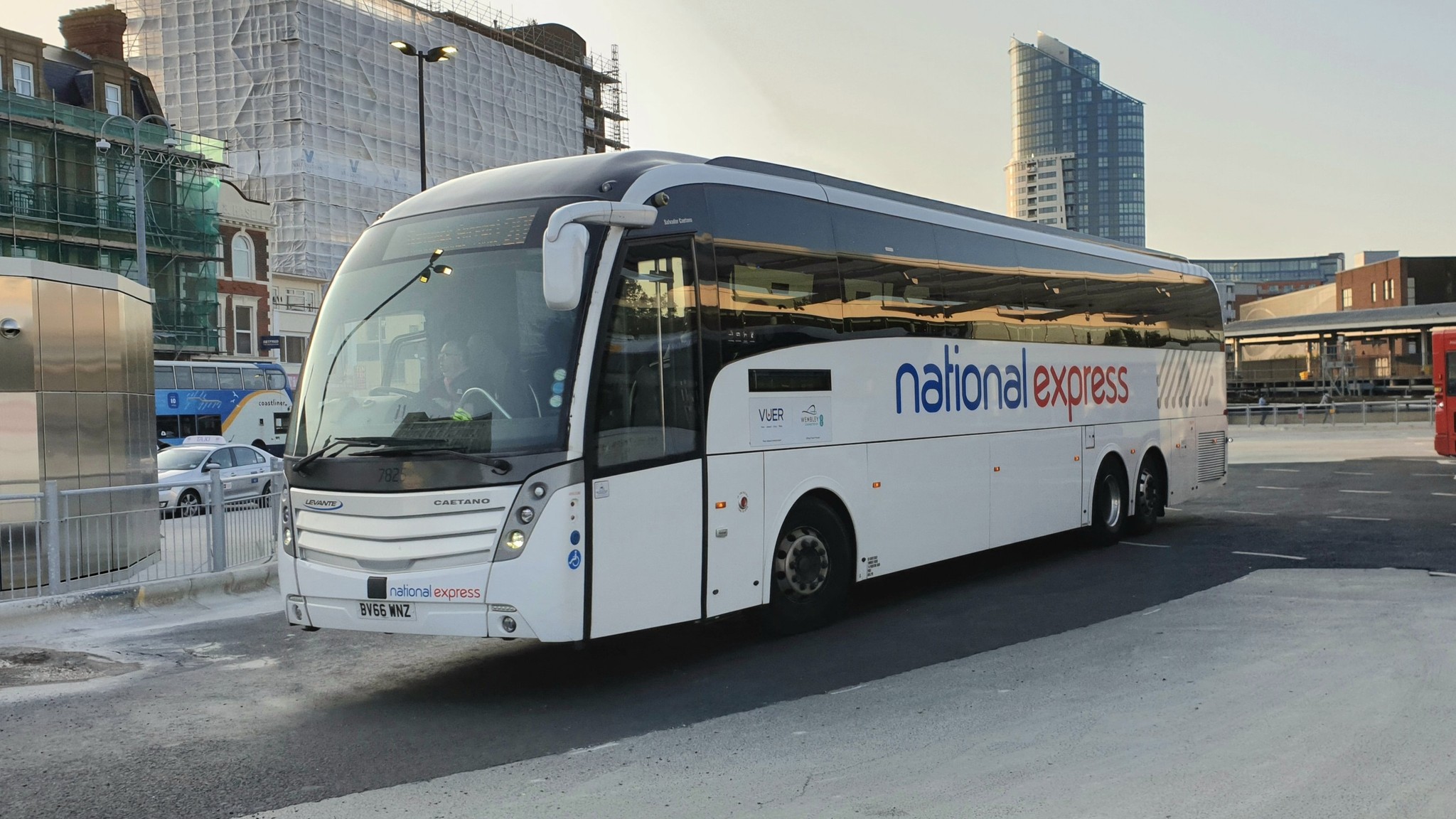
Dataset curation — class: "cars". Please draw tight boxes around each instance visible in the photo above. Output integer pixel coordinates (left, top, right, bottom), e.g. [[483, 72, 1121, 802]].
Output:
[[156, 443, 284, 518], [362, 387, 408, 409]]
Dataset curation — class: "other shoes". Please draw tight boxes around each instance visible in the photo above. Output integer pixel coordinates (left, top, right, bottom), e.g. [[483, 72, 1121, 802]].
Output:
[[1260, 424, 1266, 427]]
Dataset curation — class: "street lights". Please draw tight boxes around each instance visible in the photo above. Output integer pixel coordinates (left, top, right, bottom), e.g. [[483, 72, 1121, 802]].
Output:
[[309, 250, 452, 456], [391, 40, 458, 193]]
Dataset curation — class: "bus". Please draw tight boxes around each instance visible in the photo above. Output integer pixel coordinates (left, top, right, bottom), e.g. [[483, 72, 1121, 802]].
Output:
[[277, 150, 1233, 644], [153, 360, 294, 457], [1431, 326, 1456, 456]]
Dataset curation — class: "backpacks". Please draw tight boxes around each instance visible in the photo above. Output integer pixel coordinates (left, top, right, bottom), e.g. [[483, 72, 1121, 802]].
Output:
[[1324, 394, 1333, 407]]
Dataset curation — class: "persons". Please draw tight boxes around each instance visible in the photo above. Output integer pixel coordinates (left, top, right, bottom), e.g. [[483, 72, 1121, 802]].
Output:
[[1317, 390, 1334, 424], [1258, 393, 1269, 425], [392, 338, 493, 422]]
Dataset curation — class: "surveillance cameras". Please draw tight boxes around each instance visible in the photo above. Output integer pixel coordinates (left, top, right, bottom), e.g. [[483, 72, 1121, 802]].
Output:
[[163, 138, 178, 147], [96, 139, 111, 153]]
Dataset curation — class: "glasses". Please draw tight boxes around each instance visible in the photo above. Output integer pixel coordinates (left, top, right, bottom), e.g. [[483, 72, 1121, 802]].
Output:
[[437, 352, 457, 360]]
